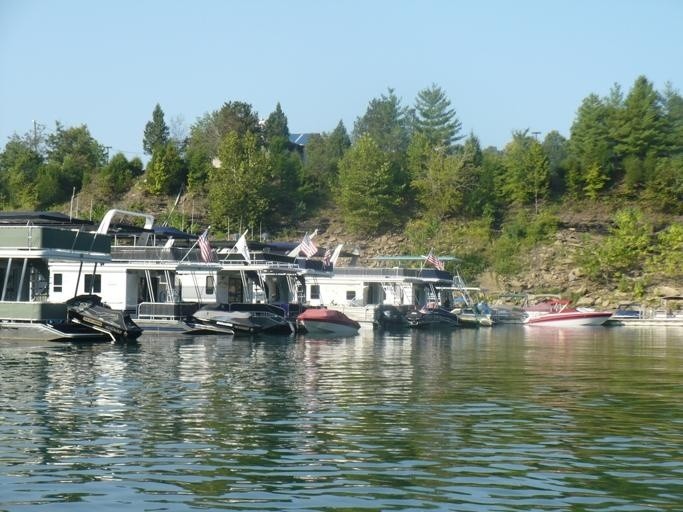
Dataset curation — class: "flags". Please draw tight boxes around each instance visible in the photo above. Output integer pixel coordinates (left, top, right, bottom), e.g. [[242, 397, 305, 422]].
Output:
[[427, 251, 443, 267], [235, 235, 251, 265], [300, 232, 318, 258], [197, 229, 213, 263]]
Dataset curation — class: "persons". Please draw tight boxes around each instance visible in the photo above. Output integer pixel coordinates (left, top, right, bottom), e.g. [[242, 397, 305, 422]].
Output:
[[667, 309, 673, 318], [477, 300, 485, 314]]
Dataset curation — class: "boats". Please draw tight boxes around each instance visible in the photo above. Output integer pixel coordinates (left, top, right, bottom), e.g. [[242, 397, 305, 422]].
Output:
[[492, 293, 527, 323], [373, 268, 497, 333], [620, 295, 683, 327], [516, 297, 613, 330], [295, 308, 361, 337], [0, 188, 310, 344], [604, 294, 647, 329]]
